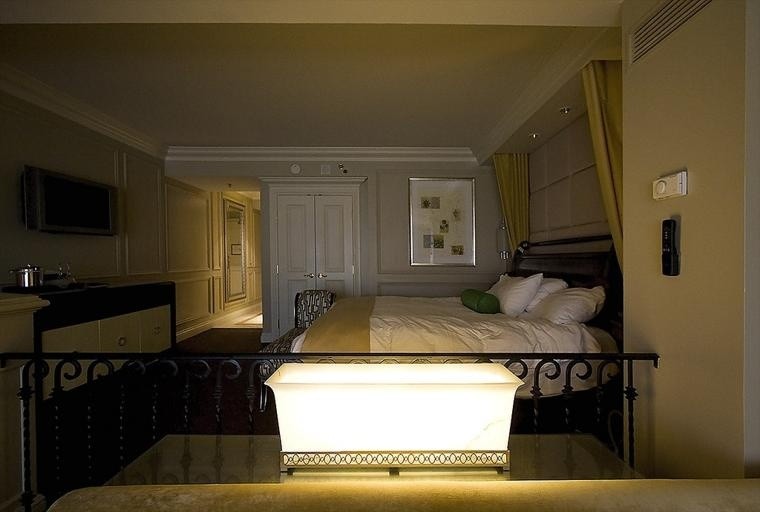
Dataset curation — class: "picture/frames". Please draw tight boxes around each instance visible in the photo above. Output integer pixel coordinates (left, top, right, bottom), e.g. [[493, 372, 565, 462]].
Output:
[[408, 176, 475, 266]]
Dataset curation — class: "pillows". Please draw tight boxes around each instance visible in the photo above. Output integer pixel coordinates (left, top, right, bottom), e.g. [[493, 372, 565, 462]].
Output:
[[528, 285, 606, 321], [460, 287, 496, 310], [526, 276, 567, 310], [485, 273, 543, 318]]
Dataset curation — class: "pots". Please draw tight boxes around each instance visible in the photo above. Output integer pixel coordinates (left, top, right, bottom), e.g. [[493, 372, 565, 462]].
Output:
[[8, 264, 41, 290]]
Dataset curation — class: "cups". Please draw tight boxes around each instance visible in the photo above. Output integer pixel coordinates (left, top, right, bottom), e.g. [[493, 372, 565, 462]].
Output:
[[72, 272, 80, 284]]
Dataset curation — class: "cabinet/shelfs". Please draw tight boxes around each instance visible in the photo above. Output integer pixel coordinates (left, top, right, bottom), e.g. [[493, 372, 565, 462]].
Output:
[[31, 283, 174, 401]]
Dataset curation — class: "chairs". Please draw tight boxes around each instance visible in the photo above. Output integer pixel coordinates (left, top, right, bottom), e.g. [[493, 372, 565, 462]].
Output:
[[292, 290, 336, 329]]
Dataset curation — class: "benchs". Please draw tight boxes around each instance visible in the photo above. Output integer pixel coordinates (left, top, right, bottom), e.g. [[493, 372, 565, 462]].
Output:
[[258, 326, 308, 412]]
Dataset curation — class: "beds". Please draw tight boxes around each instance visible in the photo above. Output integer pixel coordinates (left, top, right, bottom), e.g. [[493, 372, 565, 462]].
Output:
[[294, 233, 622, 421]]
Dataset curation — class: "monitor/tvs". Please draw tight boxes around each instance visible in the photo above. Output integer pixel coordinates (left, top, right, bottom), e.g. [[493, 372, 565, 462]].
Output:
[[20, 164, 120, 237]]
